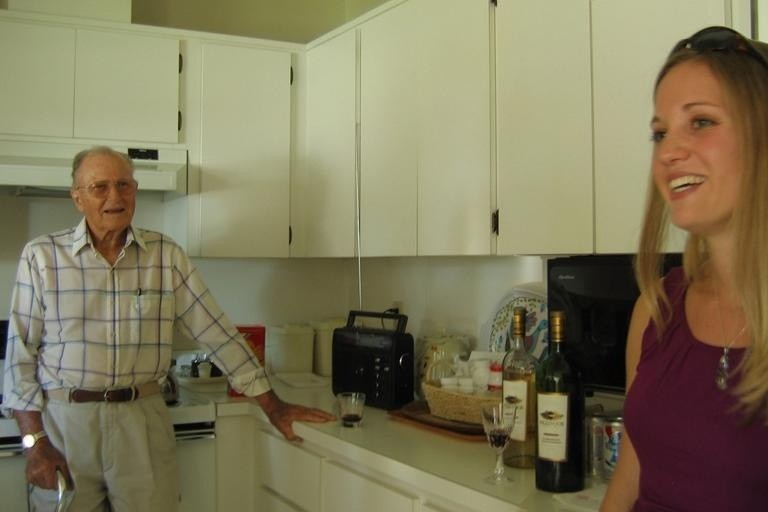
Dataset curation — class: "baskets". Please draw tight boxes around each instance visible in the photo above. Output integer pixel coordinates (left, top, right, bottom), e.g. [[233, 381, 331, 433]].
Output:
[[423, 361, 502, 427]]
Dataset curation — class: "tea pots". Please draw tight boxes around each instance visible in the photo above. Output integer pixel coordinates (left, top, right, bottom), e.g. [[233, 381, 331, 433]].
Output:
[[414, 322, 470, 401]]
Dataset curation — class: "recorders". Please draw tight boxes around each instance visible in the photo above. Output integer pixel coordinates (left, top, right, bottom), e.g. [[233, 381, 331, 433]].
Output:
[[332, 310, 414, 411]]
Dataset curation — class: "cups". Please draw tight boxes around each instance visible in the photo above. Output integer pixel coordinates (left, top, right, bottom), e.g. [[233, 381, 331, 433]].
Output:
[[335, 389, 366, 425]]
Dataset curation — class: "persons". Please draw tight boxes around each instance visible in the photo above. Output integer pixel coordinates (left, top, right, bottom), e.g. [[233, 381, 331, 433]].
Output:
[[0, 146, 337, 512], [590, 25, 768, 512]]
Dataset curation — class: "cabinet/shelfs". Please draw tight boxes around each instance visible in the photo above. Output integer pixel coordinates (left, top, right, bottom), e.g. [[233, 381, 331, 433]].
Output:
[[294, 18, 357, 260], [0, 443, 35, 511], [175, 435, 217, 511], [357, 2, 490, 260], [252, 430, 479, 512], [494, 0, 752, 255], [0, 15, 181, 144], [162, 34, 292, 259]]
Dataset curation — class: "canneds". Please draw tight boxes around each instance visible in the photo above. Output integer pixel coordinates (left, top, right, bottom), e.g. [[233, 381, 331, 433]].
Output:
[[583, 414, 607, 476], [602, 417, 626, 473]]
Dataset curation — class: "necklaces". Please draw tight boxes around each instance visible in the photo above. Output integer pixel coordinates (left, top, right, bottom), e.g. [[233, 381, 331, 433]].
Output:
[[703, 258, 751, 391]]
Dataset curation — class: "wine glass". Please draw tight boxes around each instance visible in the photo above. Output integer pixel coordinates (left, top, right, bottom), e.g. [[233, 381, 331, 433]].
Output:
[[483, 404, 519, 488]]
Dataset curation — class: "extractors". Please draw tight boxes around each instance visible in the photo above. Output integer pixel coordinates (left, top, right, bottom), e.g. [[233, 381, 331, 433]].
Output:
[[0, 0, 188, 195]]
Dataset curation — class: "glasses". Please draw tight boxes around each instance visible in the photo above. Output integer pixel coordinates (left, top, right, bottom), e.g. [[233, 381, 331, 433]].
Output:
[[663, 27, 762, 71], [79, 180, 138, 199]]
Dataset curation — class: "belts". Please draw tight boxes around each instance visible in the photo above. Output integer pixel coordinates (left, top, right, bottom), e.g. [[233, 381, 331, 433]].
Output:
[[41, 380, 161, 403]]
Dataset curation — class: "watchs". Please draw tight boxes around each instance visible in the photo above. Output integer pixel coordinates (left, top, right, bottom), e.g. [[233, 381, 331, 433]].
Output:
[[20, 430, 48, 449]]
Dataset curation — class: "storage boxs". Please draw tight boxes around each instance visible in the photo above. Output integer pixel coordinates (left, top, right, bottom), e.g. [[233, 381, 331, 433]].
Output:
[[270, 322, 315, 376], [313, 317, 366, 378]]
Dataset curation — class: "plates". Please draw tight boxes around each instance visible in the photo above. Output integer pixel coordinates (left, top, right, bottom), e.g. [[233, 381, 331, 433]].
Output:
[[477, 289, 548, 360], [276, 369, 330, 389]]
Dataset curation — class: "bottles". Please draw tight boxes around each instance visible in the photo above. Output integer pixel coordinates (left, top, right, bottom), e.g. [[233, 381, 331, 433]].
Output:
[[188, 350, 224, 379], [536, 309, 585, 494], [441, 359, 501, 395], [501, 303, 538, 466]]
